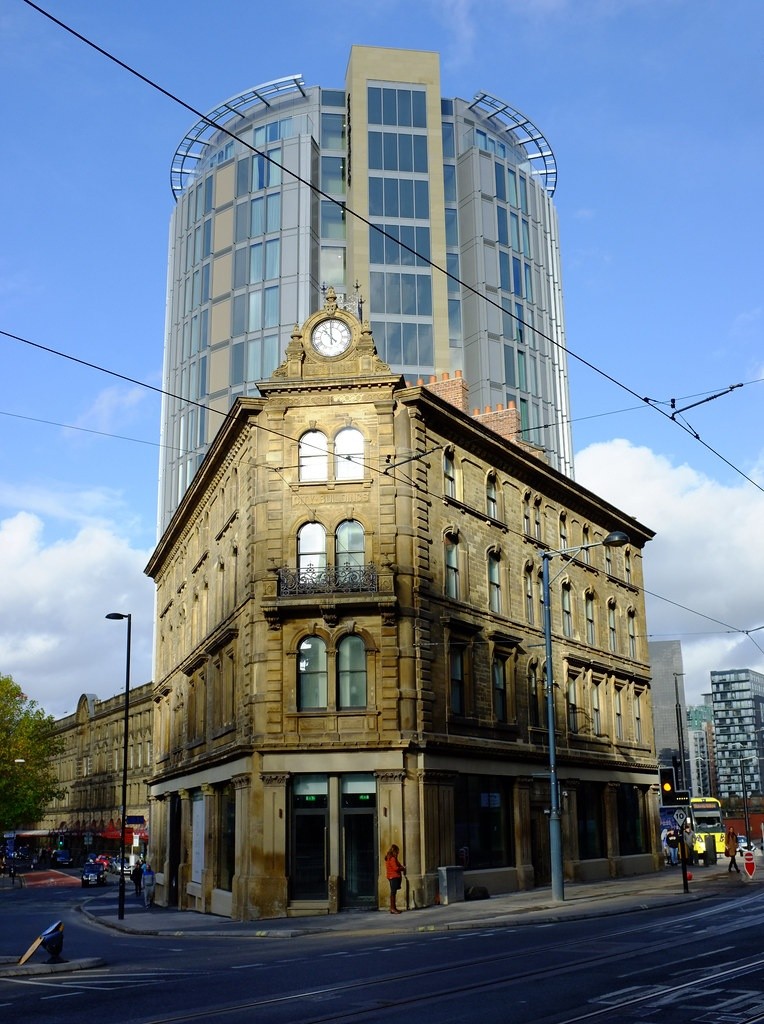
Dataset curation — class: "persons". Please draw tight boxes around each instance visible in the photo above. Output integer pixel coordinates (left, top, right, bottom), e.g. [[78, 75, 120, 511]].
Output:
[[385, 845, 405, 914], [663, 829, 678, 866], [133, 864, 144, 897], [141, 860, 146, 871], [725, 827, 741, 873], [140, 864, 156, 908], [684, 826, 699, 867]]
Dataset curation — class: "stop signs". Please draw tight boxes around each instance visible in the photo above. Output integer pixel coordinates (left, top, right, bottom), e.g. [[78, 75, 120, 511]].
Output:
[[743, 851, 754, 863]]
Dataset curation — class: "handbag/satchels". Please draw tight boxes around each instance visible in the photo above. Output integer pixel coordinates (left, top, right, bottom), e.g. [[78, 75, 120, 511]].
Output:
[[725, 849, 729, 856]]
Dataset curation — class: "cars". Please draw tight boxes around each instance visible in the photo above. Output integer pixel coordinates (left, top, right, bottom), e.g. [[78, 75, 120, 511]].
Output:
[[736, 835, 756, 853], [51, 849, 74, 869], [78, 851, 133, 875], [80, 862, 108, 887]]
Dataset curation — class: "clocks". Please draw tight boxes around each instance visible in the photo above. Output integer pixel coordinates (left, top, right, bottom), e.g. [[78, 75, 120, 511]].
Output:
[[310, 316, 353, 359]]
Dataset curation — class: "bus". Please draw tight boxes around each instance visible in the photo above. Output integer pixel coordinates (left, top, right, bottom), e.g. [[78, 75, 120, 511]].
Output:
[[658, 797, 726, 859]]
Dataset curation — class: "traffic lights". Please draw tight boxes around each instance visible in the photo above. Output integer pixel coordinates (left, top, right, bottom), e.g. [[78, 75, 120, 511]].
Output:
[[658, 767, 692, 807]]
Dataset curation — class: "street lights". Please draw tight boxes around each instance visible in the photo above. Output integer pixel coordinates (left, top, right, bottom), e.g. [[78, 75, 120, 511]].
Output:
[[104, 612, 132, 921], [673, 672, 688, 790], [543, 532, 629, 901], [740, 758, 753, 852]]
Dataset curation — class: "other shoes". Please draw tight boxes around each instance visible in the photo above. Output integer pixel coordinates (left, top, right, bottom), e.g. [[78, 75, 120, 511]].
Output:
[[728, 868, 731, 872], [737, 870, 740, 873]]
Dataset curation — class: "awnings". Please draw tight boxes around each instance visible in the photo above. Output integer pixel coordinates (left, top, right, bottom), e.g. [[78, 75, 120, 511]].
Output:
[[18, 830, 68, 838]]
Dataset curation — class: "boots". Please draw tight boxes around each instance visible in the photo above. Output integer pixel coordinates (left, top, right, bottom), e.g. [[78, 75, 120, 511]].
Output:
[[390, 895, 402, 914]]
